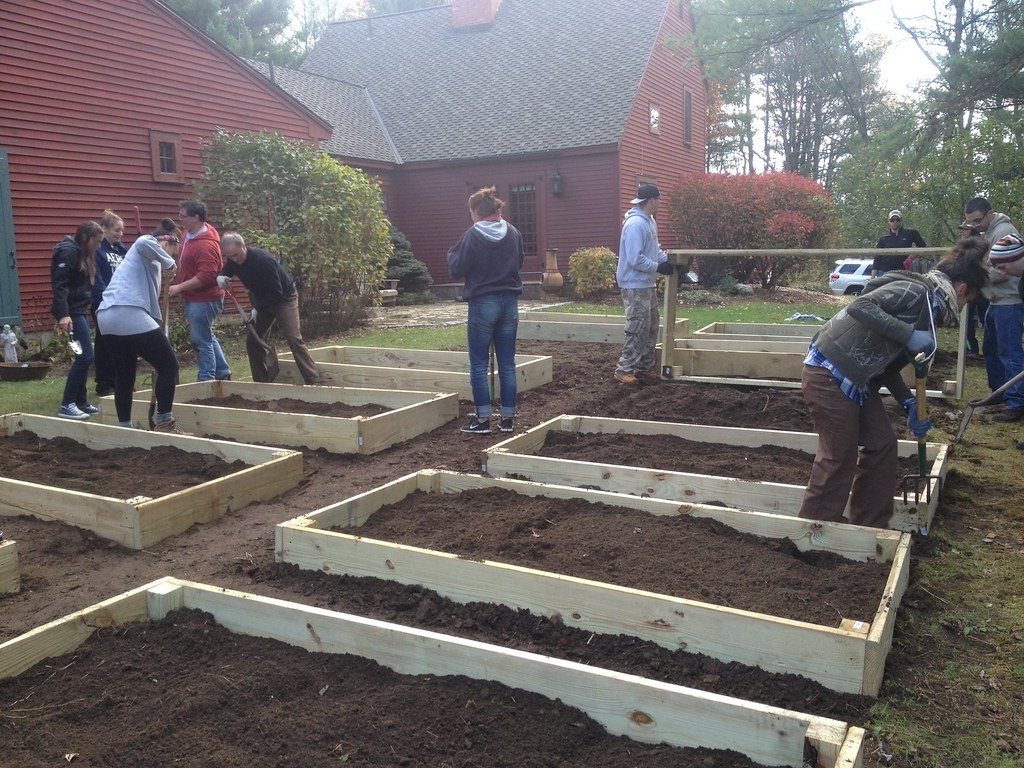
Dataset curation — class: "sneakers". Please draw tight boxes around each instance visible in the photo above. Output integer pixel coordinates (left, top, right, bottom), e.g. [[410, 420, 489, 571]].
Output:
[[154, 417, 195, 436], [460, 415, 492, 433], [497, 416, 513, 436], [58, 403, 90, 419], [80, 404, 99, 415]]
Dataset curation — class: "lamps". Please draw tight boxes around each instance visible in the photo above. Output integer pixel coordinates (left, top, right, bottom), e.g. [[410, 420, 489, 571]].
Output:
[[549, 169, 563, 193]]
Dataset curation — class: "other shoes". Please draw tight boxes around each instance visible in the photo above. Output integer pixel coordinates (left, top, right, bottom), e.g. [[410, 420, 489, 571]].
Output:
[[973, 390, 1005, 405], [966, 349, 979, 359], [614, 370, 638, 384], [95, 381, 115, 396], [634, 368, 657, 379], [992, 407, 1024, 422]]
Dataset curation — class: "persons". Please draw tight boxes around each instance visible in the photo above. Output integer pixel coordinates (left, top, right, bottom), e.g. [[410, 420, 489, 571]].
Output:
[[90, 210, 129, 397], [168, 201, 232, 382], [989, 233, 1024, 450], [965, 197, 1024, 423], [50, 221, 105, 420], [613, 185, 674, 383], [95, 218, 195, 436], [0, 325, 18, 363], [872, 210, 926, 277], [216, 233, 320, 385], [798, 237, 991, 529], [958, 219, 990, 355], [447, 188, 525, 434]]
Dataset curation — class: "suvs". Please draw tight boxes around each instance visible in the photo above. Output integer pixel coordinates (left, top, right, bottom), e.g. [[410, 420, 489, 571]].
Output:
[[826, 257, 876, 298]]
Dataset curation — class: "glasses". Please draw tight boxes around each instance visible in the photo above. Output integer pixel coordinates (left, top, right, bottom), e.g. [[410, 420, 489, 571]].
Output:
[[967, 209, 991, 224], [891, 218, 900, 222], [180, 214, 191, 220]]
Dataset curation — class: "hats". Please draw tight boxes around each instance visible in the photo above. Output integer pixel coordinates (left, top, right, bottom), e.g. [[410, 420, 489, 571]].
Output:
[[630, 184, 660, 204], [989, 232, 1024, 268], [958, 219, 973, 230], [888, 210, 901, 220]]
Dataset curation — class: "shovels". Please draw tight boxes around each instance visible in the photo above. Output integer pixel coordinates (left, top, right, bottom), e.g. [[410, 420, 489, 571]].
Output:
[[226, 288, 280, 383], [148, 266, 173, 431], [957, 370, 1024, 442], [67, 331, 82, 355]]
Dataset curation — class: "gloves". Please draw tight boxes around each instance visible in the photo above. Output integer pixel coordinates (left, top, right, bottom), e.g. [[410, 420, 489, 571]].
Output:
[[908, 330, 934, 358], [656, 260, 682, 275], [903, 256, 913, 270], [244, 309, 258, 325], [217, 276, 231, 290], [902, 396, 930, 436]]
